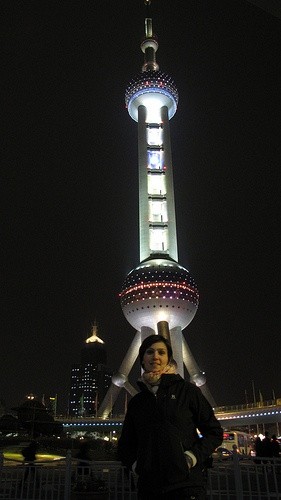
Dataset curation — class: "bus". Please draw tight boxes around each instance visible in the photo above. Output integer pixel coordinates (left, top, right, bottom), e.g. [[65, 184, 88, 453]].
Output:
[[216, 431, 256, 457]]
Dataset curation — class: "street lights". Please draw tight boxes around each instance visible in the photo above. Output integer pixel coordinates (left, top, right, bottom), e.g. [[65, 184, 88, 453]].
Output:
[[25, 392, 37, 438]]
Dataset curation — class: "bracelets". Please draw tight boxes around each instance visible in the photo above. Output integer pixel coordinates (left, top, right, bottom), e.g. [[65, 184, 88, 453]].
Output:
[[185, 454, 193, 466]]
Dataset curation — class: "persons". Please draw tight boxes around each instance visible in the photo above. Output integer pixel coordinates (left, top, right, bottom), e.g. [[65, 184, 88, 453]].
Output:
[[254, 431, 280, 472], [73, 441, 95, 490], [18, 439, 41, 492], [118, 334, 224, 500]]
